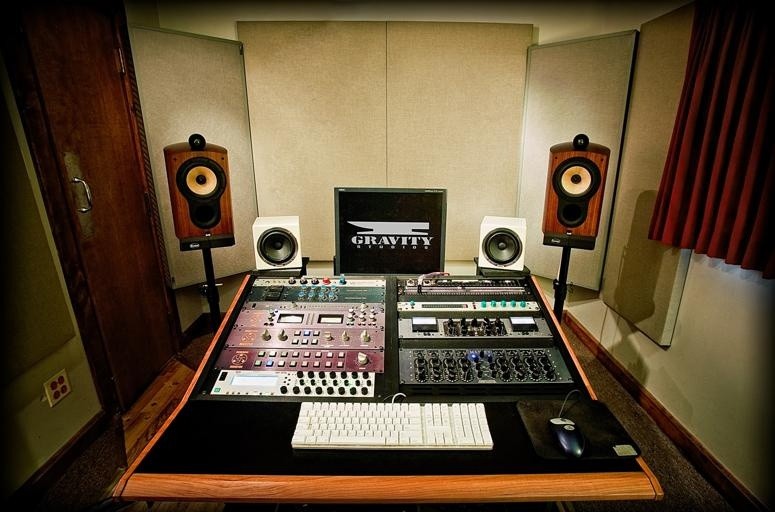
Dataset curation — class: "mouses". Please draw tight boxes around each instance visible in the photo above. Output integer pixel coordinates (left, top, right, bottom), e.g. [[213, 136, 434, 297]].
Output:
[[549, 417, 585, 457]]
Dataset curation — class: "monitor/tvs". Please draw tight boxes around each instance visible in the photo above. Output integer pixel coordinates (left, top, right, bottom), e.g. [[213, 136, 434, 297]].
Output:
[[334, 187, 447, 276]]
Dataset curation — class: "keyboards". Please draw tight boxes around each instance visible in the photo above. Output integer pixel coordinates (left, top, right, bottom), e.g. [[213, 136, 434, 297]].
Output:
[[291, 402, 495, 451]]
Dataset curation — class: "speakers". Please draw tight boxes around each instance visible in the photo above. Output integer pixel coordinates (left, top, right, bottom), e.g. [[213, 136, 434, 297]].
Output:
[[252, 216, 303, 270], [478, 216, 526, 271], [542, 143, 611, 237], [163, 142, 234, 240]]
